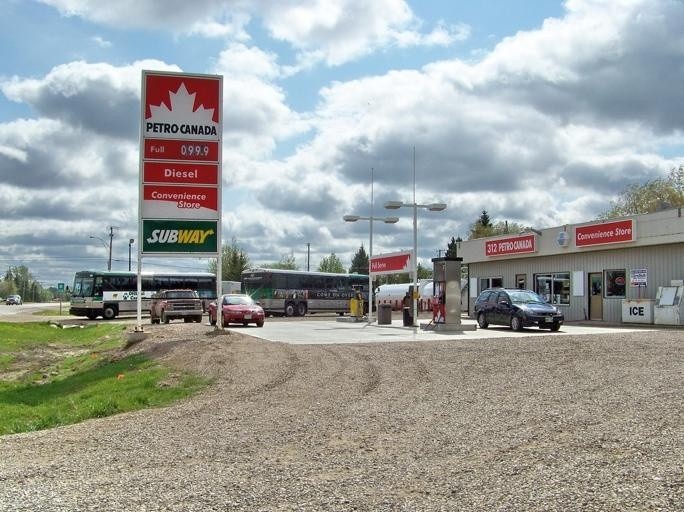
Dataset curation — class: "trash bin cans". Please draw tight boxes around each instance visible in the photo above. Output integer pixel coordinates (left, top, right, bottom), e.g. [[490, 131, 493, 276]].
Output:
[[378, 303, 391, 324]]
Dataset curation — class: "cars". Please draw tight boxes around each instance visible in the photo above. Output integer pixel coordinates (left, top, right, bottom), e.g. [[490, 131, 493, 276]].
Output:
[[473, 287, 564, 331], [6, 294, 22, 305], [208, 293, 265, 327]]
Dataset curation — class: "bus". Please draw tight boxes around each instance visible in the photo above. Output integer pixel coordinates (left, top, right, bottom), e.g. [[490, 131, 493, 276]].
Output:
[[240, 268, 376, 318], [66, 271, 217, 319]]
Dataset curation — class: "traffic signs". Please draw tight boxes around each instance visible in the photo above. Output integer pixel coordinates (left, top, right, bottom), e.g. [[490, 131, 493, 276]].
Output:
[[58, 283, 64, 293]]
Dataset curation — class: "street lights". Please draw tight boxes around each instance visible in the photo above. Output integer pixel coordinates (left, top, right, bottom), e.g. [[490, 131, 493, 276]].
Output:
[[342, 214, 398, 321], [89, 226, 114, 271], [384, 200, 447, 324], [128, 239, 135, 271]]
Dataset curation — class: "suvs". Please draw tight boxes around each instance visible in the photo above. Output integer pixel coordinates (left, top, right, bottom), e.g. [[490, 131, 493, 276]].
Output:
[[151, 288, 204, 324]]
